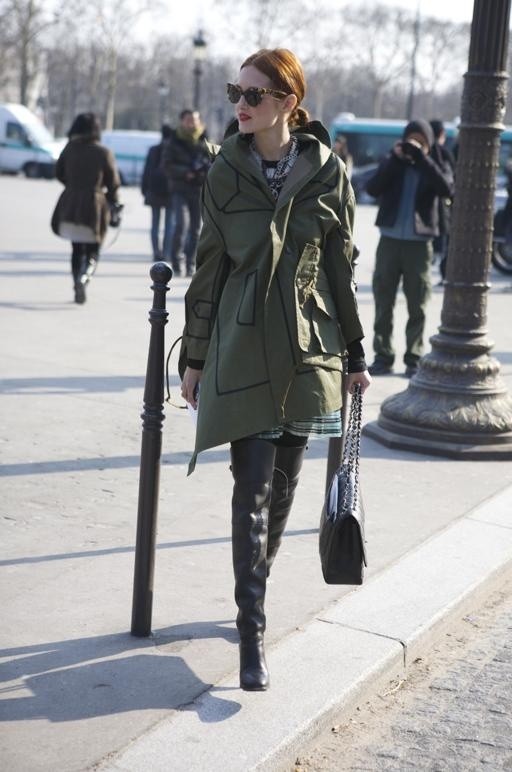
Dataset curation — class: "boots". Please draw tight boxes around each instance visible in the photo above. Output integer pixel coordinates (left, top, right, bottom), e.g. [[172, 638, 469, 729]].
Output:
[[71, 254, 96, 304], [231, 440, 307, 692]]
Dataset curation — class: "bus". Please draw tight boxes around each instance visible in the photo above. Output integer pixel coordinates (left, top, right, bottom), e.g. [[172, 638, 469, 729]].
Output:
[[327, 112, 511, 207]]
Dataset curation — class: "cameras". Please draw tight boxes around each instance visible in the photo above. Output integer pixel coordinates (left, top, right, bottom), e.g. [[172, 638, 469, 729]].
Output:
[[402, 143, 416, 155]]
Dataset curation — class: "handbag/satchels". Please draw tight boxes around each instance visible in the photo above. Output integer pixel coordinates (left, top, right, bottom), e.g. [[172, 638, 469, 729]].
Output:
[[318, 461, 366, 585]]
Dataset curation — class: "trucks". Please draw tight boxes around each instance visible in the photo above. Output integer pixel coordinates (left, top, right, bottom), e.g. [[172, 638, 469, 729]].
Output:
[[1, 102, 60, 180], [94, 128, 167, 187]]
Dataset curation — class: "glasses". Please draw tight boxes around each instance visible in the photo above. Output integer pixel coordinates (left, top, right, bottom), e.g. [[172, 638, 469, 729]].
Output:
[[225, 81, 287, 107]]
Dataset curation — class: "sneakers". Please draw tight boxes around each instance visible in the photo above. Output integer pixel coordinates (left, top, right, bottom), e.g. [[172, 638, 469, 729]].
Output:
[[369, 361, 395, 375]]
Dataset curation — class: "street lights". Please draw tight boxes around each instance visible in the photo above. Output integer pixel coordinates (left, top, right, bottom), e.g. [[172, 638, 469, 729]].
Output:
[[191, 28, 208, 115]]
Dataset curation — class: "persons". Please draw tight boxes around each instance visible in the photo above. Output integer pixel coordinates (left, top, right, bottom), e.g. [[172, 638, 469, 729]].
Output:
[[162, 107, 212, 278], [140, 125, 182, 268], [49, 111, 120, 306], [176, 46, 375, 691], [425, 115, 451, 288], [362, 116, 454, 380], [331, 132, 353, 190]]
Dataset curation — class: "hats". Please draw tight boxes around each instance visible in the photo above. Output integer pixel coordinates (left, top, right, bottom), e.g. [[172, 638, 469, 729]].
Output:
[[402, 118, 434, 154]]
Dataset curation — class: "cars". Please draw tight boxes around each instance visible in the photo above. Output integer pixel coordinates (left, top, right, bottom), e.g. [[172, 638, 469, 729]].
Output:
[[490, 171, 511, 278]]
